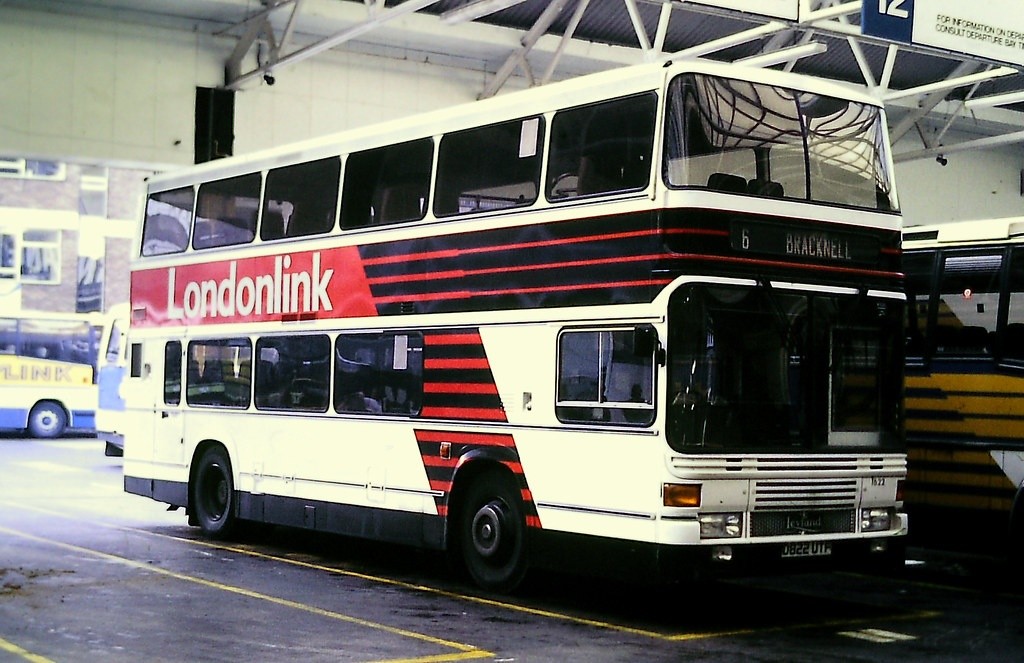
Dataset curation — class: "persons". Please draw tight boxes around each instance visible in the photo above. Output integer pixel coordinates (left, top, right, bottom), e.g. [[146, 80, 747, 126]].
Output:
[[338, 373, 382, 413]]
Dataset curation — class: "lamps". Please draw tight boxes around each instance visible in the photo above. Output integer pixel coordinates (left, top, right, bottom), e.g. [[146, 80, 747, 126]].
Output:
[[264, 71, 275, 85], [936, 153, 948, 166]]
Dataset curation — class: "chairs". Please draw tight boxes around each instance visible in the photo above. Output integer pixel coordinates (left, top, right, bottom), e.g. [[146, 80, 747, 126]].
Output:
[[172, 359, 420, 417], [146, 158, 783, 252], [930, 322, 1024, 362]]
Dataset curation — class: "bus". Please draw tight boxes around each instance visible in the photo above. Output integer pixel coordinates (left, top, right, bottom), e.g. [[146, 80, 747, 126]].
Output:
[[830, 215, 1024, 574], [97, 301, 129, 458], [121, 51, 916, 601], [0, 309, 107, 440]]
